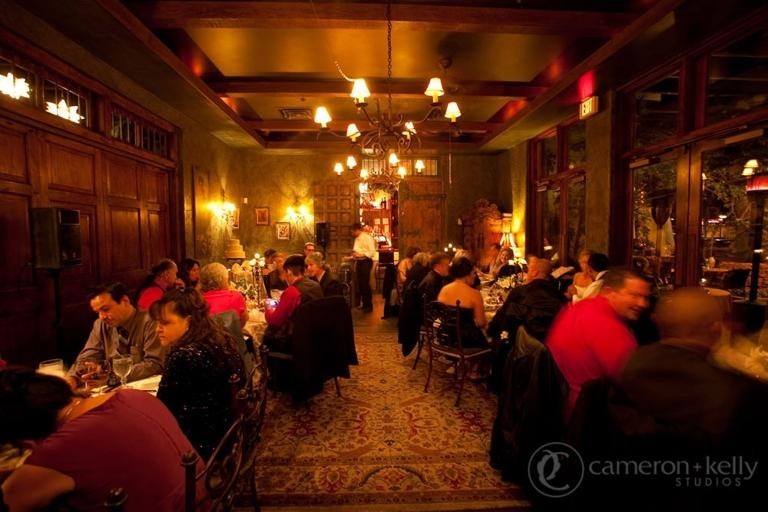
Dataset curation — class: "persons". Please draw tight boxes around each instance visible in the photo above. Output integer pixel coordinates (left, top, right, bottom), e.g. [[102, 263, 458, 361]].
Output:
[[342, 224, 375, 315]]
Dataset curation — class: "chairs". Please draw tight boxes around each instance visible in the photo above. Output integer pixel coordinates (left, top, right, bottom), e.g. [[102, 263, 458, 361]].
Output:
[[390, 254, 565, 482], [0, 254, 356, 509]]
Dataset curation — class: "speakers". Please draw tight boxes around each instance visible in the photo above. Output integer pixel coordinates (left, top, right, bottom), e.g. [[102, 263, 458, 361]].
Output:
[[316, 222, 330, 246], [32, 207, 82, 270]]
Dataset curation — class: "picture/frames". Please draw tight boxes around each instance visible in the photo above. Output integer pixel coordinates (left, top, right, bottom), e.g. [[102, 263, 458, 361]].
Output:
[[254, 207, 270, 227], [274, 221, 291, 241], [192, 164, 210, 260]]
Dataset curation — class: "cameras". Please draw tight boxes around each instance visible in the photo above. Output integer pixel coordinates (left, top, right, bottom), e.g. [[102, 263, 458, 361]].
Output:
[[269, 299, 279, 308]]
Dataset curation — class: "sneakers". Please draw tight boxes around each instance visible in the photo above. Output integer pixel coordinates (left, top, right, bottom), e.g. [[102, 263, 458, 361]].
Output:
[[437, 356, 452, 365]]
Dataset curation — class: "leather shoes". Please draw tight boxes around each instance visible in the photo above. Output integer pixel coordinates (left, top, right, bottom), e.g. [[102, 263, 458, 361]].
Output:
[[358, 306, 373, 312]]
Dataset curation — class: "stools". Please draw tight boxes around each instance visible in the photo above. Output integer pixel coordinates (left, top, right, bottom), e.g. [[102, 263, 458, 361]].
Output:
[[373, 262, 393, 291]]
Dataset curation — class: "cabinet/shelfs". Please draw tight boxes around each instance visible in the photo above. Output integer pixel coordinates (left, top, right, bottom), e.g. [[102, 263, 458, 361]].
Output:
[[471, 207, 504, 269]]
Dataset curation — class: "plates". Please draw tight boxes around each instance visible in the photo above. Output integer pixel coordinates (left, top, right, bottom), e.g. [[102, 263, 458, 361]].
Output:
[[483, 297, 504, 306]]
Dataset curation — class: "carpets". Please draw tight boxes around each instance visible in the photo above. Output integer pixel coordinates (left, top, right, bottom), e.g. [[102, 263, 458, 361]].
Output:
[[222, 331, 544, 510]]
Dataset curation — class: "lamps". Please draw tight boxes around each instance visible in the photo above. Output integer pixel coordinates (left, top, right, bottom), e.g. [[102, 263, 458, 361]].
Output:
[[307, 0, 462, 207]]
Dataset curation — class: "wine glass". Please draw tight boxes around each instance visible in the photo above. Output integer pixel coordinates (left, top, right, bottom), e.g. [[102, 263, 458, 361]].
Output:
[[40, 354, 134, 394]]
